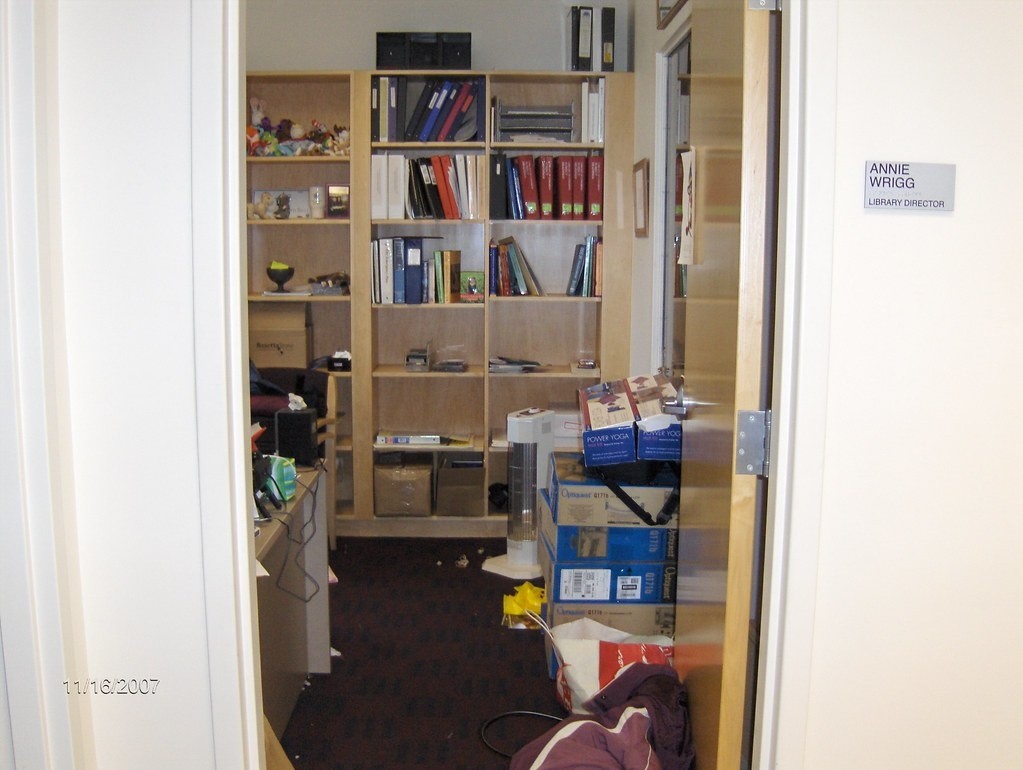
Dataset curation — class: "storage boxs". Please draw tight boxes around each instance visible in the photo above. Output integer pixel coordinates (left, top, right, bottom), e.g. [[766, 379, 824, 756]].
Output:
[[248, 302, 313, 369], [539, 373, 683, 681], [436, 454, 485, 516], [374, 452, 433, 516]]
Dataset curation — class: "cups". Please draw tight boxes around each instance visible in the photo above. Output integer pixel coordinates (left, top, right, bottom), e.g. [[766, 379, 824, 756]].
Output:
[[309, 186, 325, 219]]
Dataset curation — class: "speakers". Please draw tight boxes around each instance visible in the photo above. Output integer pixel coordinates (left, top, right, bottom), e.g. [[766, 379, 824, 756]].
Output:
[[274, 408, 318, 468]]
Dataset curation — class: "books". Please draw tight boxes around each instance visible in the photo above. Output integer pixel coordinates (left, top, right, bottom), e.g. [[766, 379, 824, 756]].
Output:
[[371, 74, 486, 142], [261, 291, 313, 297], [488, 355, 541, 374], [489, 428, 509, 448], [371, 236, 484, 304], [488, 235, 541, 297], [377, 450, 406, 469], [579, 374, 677, 431], [490, 76, 605, 144], [561, 5, 616, 72], [566, 235, 603, 298], [373, 430, 475, 450], [451, 460, 483, 468], [489, 149, 604, 221], [371, 154, 485, 219]]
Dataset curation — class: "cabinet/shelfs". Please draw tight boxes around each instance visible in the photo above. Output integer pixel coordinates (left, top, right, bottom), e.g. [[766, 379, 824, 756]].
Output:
[[245, 67, 636, 538]]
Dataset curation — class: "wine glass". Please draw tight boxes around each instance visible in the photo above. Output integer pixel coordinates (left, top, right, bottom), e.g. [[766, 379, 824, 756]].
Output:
[[266, 267, 294, 293]]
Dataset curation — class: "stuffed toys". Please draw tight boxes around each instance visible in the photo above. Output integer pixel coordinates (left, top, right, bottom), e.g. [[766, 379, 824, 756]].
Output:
[[246, 191, 291, 220], [245, 97, 350, 157]]
